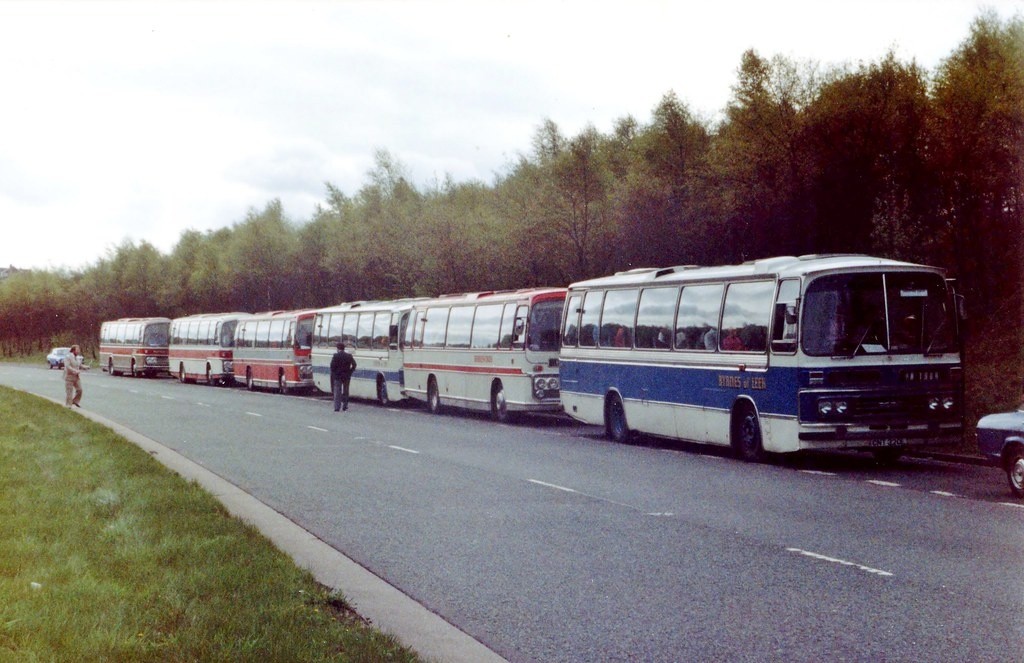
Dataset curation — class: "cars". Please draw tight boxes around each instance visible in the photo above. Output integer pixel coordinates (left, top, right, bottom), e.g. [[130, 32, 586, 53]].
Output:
[[47, 347, 84, 370], [974, 410, 1024, 499]]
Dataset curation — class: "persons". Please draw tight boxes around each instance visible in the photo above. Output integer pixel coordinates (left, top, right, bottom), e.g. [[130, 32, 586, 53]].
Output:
[[593, 321, 746, 352], [64, 344, 88, 407], [329, 343, 357, 411]]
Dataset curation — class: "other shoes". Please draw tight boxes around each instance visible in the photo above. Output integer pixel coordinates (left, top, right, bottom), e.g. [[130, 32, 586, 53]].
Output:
[[343, 407, 349, 410], [335, 408, 339, 411], [72, 402, 80, 407]]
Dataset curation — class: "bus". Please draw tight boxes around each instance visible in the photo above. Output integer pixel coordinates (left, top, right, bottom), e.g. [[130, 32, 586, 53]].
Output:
[[100, 317, 172, 379], [168, 312, 252, 387], [232, 307, 320, 395], [403, 286, 570, 424], [311, 297, 430, 408], [557, 252, 971, 465]]
